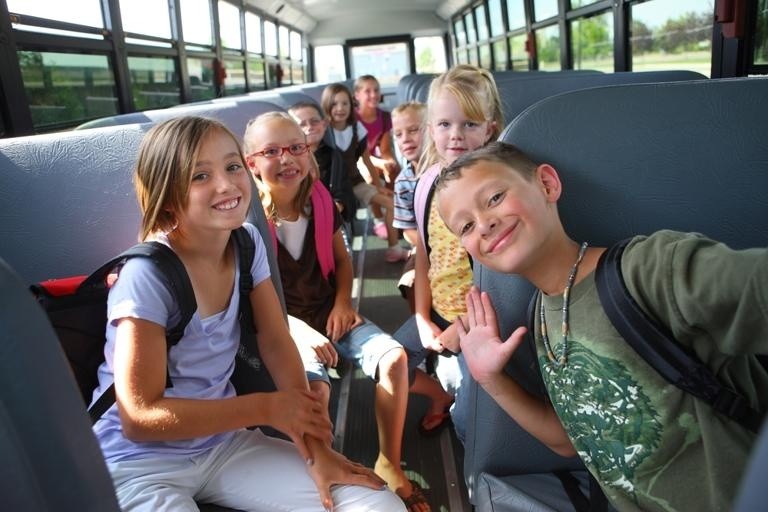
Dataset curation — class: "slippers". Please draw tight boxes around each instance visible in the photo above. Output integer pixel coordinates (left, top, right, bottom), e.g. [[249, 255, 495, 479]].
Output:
[[394, 479, 432, 512], [421, 405, 451, 435]]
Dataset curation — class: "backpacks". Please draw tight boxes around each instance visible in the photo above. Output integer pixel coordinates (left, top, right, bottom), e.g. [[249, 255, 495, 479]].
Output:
[[23, 226, 255, 432]]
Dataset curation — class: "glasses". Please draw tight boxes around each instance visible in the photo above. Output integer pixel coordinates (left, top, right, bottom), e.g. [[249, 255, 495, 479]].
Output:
[[246, 142, 309, 158]]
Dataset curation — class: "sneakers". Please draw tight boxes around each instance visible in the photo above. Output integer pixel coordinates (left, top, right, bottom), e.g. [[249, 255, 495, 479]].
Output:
[[385, 245, 414, 263], [374, 220, 391, 240]]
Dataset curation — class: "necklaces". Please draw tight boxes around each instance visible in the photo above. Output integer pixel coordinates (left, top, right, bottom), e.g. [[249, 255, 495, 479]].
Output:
[[539, 241, 588, 367]]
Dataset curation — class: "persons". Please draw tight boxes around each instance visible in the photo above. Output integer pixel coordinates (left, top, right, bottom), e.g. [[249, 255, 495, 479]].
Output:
[[434, 140, 766, 511], [84, 115, 408, 512], [390, 100, 426, 315], [320, 82, 410, 264], [351, 75, 401, 240], [391, 62, 506, 434], [287, 103, 358, 222], [241, 110, 433, 512]]
